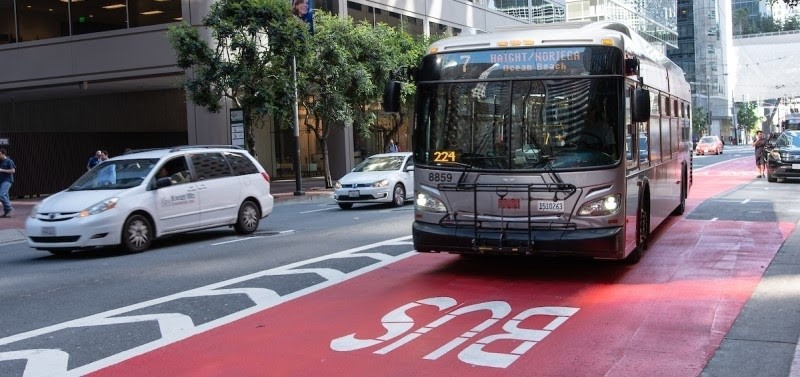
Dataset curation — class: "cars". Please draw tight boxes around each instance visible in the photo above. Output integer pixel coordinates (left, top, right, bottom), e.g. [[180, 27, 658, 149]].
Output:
[[765, 130, 800, 182], [334, 151, 415, 210], [695, 134, 724, 155]]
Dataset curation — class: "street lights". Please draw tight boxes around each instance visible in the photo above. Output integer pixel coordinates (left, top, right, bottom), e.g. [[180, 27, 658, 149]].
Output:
[[708, 73, 729, 137]]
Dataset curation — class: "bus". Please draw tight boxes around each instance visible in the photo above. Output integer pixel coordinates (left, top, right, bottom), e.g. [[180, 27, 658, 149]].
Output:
[[382, 19, 693, 266], [782, 113, 800, 131]]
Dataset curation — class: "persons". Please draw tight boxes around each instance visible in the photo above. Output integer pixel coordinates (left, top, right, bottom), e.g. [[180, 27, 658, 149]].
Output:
[[152, 165, 169, 187], [387, 139, 401, 153], [572, 96, 616, 156], [292, 0, 308, 21], [0, 149, 16, 218], [87, 149, 116, 185], [753, 131, 767, 178]]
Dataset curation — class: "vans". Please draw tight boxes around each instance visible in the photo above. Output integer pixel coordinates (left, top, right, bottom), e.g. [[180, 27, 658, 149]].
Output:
[[24, 144, 273, 254]]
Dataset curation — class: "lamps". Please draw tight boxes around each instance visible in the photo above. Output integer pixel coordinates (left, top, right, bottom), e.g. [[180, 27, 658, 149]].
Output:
[[80, 81, 88, 96]]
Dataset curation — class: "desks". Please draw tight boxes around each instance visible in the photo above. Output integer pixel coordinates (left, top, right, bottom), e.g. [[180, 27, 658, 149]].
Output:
[[301, 164, 318, 176]]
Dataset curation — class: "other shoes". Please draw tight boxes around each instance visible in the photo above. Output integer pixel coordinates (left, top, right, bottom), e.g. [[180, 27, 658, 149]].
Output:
[[756, 175, 761, 178], [762, 175, 765, 179], [7, 209, 15, 217], [0, 214, 8, 218]]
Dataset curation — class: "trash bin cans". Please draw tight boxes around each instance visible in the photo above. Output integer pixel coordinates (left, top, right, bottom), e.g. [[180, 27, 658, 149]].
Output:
[[733, 138, 736, 145]]
